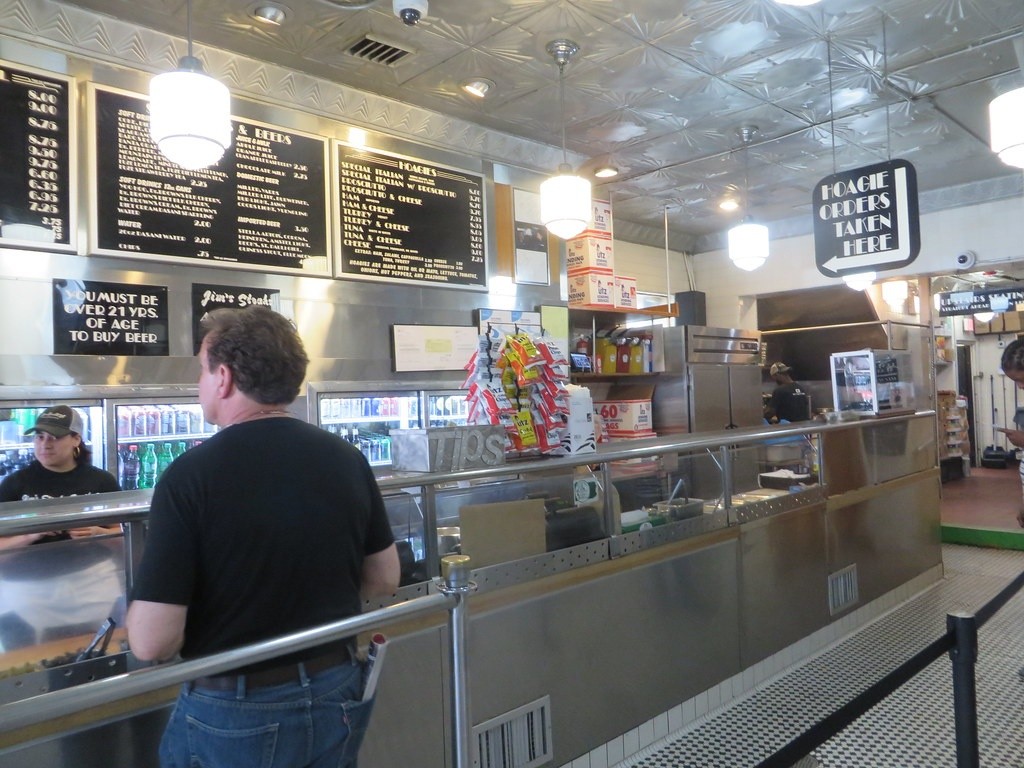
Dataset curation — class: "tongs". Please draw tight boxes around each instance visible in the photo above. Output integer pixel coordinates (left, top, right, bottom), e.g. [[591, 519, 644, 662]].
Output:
[[75, 618, 116, 665], [667, 479, 690, 507]]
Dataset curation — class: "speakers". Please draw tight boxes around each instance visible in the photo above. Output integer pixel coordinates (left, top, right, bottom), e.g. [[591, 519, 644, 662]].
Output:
[[675, 291, 707, 327]]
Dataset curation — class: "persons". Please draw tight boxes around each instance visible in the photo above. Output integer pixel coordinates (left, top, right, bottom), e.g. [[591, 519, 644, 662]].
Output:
[[125, 306, 403, 768], [763, 362, 809, 421], [1001, 340, 1024, 681], [0, 406, 123, 548]]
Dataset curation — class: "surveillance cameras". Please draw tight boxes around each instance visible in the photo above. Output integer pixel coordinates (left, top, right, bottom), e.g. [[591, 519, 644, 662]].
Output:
[[393, 0, 429, 26], [955, 251, 975, 270]]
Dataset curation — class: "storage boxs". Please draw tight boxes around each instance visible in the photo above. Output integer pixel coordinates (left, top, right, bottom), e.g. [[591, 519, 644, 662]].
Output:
[[566, 199, 638, 311], [652, 498, 703, 524], [601, 432, 658, 480], [990, 312, 1004, 333], [973, 313, 990, 334], [590, 383, 655, 436], [1004, 311, 1024, 332]]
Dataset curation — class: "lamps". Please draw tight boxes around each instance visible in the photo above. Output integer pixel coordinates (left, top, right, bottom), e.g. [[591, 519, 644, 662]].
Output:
[[728, 125, 769, 271], [988, 86, 1024, 168], [538, 39, 591, 239], [146, 0, 232, 171]]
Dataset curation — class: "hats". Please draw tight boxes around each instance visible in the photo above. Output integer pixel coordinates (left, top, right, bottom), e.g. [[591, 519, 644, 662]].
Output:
[[770, 362, 792, 375], [25, 405, 85, 439]]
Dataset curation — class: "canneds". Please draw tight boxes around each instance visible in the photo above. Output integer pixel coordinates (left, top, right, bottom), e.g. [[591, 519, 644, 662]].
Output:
[[320, 398, 419, 463], [117, 405, 217, 437], [431, 396, 473, 426]]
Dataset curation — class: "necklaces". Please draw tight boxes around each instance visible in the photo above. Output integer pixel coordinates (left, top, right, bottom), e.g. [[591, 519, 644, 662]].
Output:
[[243, 409, 289, 417]]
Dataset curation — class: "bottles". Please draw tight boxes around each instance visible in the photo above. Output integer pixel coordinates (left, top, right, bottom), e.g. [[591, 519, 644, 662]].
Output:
[[319, 425, 362, 458], [1, 440, 216, 502]]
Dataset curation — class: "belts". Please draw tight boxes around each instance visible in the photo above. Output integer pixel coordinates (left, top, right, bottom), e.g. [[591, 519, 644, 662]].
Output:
[[192, 636, 358, 692]]
